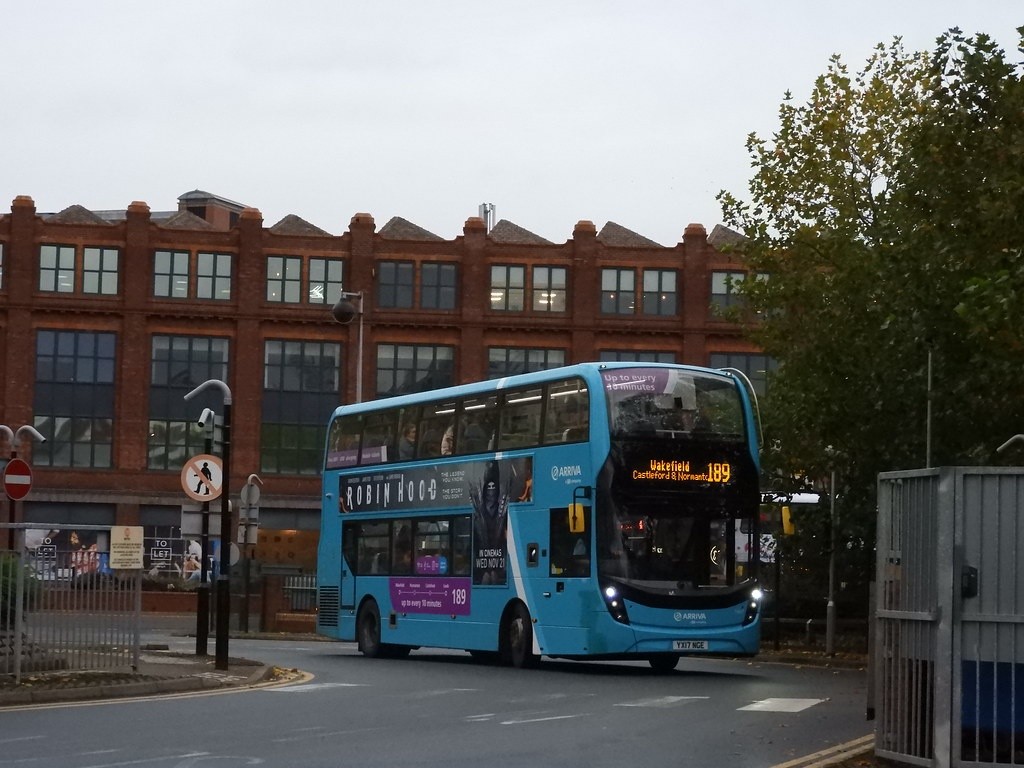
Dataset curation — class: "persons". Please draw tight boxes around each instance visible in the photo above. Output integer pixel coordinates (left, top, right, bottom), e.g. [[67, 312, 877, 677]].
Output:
[[184, 552, 211, 582], [398, 423, 416, 460], [393, 545, 412, 575], [25, 528, 100, 578], [440, 413, 468, 455]]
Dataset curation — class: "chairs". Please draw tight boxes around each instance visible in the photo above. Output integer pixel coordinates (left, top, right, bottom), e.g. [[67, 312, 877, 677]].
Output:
[[370, 552, 477, 577], [369, 422, 580, 465]]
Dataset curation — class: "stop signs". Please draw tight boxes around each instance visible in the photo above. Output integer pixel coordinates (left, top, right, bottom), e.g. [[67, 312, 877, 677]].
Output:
[[2, 458, 33, 501]]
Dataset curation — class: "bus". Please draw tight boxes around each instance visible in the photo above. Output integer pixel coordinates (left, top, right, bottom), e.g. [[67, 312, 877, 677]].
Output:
[[314, 358, 764, 674]]
